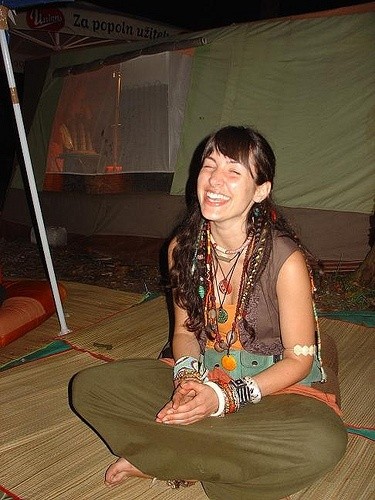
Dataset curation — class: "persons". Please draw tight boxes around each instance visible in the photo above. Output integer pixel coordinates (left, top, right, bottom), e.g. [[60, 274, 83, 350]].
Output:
[[72, 126, 348, 500]]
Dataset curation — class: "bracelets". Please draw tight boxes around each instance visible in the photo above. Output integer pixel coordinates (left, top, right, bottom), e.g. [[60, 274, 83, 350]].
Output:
[[205, 376, 262, 418], [173, 356, 205, 390]]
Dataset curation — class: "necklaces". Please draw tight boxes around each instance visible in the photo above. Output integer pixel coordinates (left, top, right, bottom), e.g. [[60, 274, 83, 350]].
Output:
[[204, 223, 256, 369]]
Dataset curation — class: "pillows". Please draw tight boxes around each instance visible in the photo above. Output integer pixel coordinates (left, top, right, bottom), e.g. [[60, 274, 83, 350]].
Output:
[[0, 276, 68, 348]]
